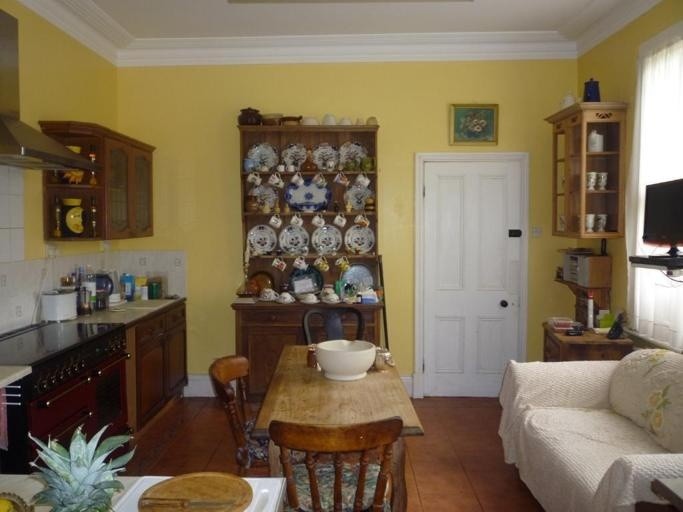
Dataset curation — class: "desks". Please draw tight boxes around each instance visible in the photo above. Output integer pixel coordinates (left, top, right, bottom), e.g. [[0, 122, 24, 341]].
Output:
[[250, 344, 422, 512]]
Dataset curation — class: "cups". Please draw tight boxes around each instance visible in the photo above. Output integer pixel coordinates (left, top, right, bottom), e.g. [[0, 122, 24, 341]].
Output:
[[258, 287, 339, 303], [238, 107, 377, 126], [246, 171, 371, 188], [271, 256, 351, 273], [269, 211, 369, 228], [276, 164, 296, 173], [586, 213, 608, 232]]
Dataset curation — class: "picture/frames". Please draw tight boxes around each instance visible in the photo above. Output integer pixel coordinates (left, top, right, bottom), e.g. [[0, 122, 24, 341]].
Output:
[[447, 103, 499, 146]]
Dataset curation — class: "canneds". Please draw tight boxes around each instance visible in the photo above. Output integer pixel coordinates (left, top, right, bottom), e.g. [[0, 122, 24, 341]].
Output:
[[307, 349, 316, 367], [96, 289, 106, 310], [140, 285, 148, 301]]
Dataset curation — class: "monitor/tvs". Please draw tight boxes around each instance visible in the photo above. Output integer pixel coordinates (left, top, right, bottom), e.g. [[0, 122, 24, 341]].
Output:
[[643, 178, 683, 258]]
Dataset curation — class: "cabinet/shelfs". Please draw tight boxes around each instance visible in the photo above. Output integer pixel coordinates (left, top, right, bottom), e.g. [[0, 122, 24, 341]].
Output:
[[545, 102, 624, 240], [543, 325, 633, 363], [230, 297, 385, 399], [40, 122, 154, 239], [52, 298, 187, 439], [236, 124, 380, 292]]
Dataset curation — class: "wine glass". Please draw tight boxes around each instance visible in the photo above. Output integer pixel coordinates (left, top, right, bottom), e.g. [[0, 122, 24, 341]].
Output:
[[584, 171, 608, 191]]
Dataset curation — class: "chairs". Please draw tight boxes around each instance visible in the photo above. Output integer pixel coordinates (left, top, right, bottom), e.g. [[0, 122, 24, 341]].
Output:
[[265, 415, 406, 512], [208, 356, 269, 478], [303, 308, 364, 345]]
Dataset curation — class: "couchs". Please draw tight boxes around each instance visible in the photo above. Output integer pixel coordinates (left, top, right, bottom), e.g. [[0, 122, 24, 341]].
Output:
[[497, 349, 682, 512]]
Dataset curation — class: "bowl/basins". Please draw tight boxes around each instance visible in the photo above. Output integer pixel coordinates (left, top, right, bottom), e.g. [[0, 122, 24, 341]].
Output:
[[315, 340, 377, 382]]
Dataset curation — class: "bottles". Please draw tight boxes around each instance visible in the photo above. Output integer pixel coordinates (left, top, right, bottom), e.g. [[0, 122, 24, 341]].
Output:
[[86, 196, 98, 238], [306, 344, 317, 367], [88, 143, 100, 185], [51, 194, 61, 239]]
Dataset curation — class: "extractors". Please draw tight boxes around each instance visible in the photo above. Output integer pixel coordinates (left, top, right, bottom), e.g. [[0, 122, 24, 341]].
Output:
[[1, 9, 104, 174]]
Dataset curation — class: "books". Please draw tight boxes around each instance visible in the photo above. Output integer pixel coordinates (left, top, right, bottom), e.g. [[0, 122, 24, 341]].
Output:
[[548, 317, 574, 333]]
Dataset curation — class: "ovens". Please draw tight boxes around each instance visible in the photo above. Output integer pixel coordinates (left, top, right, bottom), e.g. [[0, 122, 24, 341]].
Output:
[[23, 352, 132, 463]]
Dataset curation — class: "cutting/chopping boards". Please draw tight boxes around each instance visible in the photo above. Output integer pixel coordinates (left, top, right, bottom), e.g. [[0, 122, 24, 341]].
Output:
[[111, 472, 284, 512]]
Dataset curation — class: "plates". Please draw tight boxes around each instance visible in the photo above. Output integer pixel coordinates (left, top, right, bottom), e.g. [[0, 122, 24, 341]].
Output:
[[249, 263, 376, 295], [246, 224, 374, 255], [248, 178, 371, 211], [246, 141, 368, 172]]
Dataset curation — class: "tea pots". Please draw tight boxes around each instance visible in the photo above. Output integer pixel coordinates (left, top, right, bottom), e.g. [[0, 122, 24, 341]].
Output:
[[582, 76, 601, 102], [88, 274, 114, 311]]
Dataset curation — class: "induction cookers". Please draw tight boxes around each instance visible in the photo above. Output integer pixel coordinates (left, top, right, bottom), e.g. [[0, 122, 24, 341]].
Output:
[[0, 321, 128, 398]]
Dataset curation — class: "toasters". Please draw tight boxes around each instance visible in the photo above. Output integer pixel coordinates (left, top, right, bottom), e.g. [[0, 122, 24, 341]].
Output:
[[39, 287, 79, 323]]
